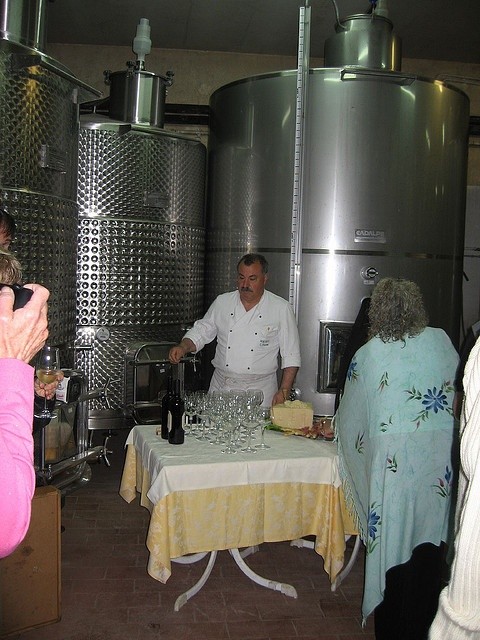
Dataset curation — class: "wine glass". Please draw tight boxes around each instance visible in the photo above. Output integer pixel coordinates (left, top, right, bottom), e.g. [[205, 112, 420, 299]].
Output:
[[34, 345, 60, 420], [181, 388, 275, 455]]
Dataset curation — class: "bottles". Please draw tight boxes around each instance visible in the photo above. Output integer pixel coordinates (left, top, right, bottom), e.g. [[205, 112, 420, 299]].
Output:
[[161, 377, 174, 440], [167, 379, 186, 444]]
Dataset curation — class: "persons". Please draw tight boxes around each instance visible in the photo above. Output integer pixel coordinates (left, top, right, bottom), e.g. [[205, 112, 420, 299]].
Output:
[[453, 320, 480, 422], [335, 277, 461, 639], [169, 253, 302, 408], [0, 209, 16, 251], [0, 252, 64, 438], [0, 283, 51, 559]]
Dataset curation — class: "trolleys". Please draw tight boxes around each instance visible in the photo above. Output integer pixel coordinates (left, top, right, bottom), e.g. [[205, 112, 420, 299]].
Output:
[[34, 367, 121, 486]]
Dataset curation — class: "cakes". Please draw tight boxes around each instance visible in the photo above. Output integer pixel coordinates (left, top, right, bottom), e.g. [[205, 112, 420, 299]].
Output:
[[271, 401, 315, 433]]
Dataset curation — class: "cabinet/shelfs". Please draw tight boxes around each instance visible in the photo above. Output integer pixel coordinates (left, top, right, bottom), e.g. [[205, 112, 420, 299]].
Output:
[[0, 485, 63, 639]]
